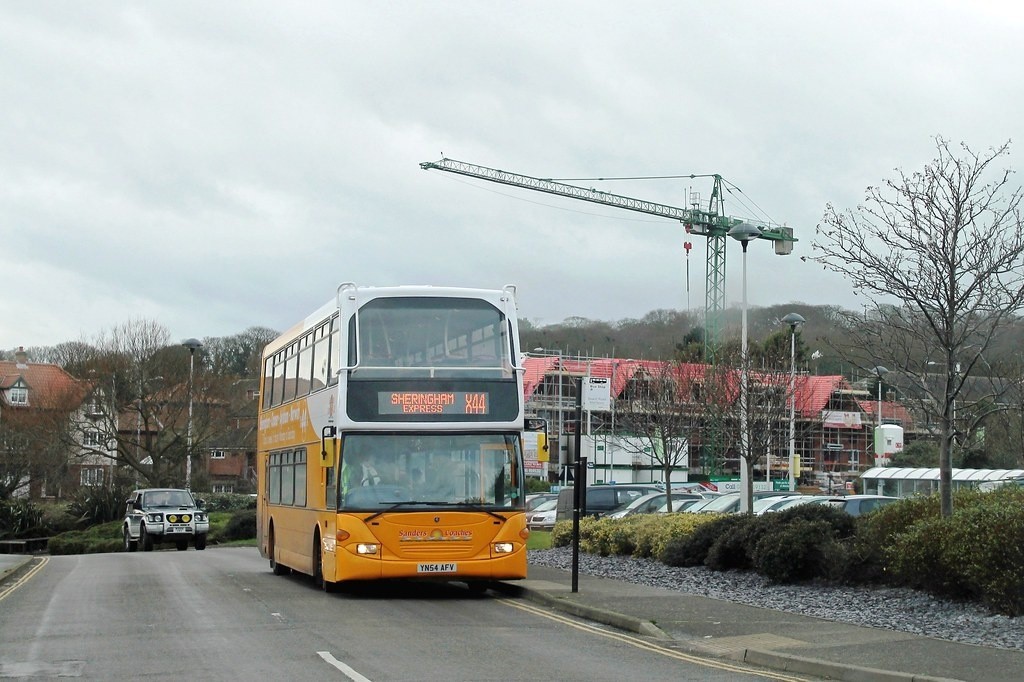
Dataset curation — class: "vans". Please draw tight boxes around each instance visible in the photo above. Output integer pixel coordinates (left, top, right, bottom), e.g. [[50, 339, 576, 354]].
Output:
[[555, 486, 665, 524]]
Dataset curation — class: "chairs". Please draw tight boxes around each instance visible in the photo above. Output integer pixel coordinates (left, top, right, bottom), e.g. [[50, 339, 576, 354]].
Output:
[[364, 353, 396, 378], [472, 354, 496, 377], [441, 353, 469, 376]]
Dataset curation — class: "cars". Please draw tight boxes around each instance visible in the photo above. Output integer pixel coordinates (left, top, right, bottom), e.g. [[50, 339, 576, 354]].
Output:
[[821, 495, 900, 517], [525, 498, 558, 531], [608, 491, 734, 519], [738, 496, 811, 515], [777, 496, 840, 512], [697, 490, 801, 515], [505, 494, 558, 512], [529, 510, 557, 532]]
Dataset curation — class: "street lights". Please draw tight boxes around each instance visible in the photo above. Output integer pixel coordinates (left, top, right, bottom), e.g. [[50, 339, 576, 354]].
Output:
[[728, 223, 763, 513], [182, 338, 203, 493], [928, 361, 956, 445], [871, 366, 888, 496], [534, 348, 562, 491], [781, 312, 806, 492]]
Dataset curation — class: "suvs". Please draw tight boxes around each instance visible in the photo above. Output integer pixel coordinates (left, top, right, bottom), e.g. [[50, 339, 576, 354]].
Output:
[[122, 487, 210, 552]]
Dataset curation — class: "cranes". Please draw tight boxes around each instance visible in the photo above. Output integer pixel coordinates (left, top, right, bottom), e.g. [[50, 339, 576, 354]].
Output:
[[420, 151, 798, 477]]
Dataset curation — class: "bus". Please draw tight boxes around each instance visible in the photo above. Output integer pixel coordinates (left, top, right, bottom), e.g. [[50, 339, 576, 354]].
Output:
[[256, 282, 551, 592]]
